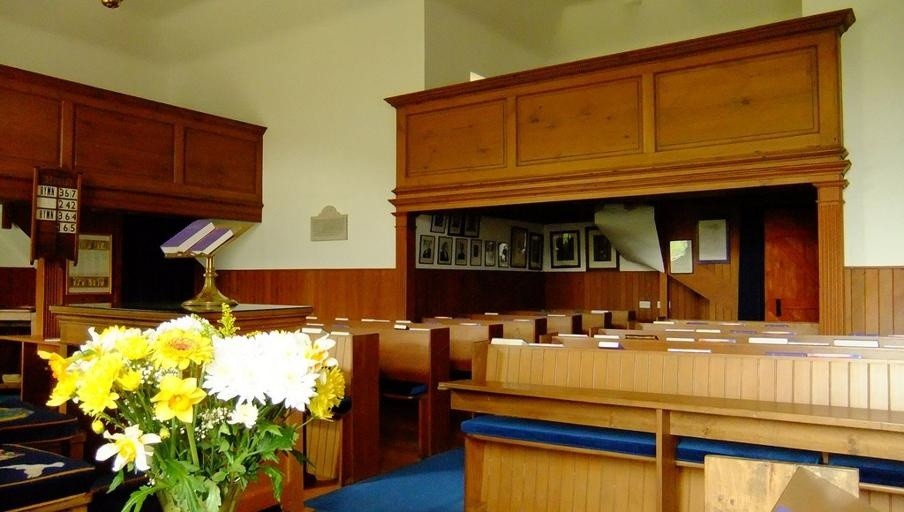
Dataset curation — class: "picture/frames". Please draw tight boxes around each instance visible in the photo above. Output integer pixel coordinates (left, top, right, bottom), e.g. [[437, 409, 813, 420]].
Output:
[[416, 215, 620, 272], [668, 219, 731, 275]]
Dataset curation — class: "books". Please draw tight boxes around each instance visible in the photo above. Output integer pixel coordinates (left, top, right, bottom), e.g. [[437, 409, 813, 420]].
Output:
[[491, 310, 624, 349], [626, 321, 904, 358], [188, 227, 234, 256], [302, 312, 499, 338], [158, 218, 216, 254]]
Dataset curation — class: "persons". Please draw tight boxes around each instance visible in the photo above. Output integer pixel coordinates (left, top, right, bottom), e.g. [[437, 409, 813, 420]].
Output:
[[418, 214, 509, 265], [555, 236, 571, 261]]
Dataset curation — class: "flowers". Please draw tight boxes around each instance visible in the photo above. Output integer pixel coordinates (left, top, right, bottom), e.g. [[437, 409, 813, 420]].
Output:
[[36, 300, 348, 512]]
